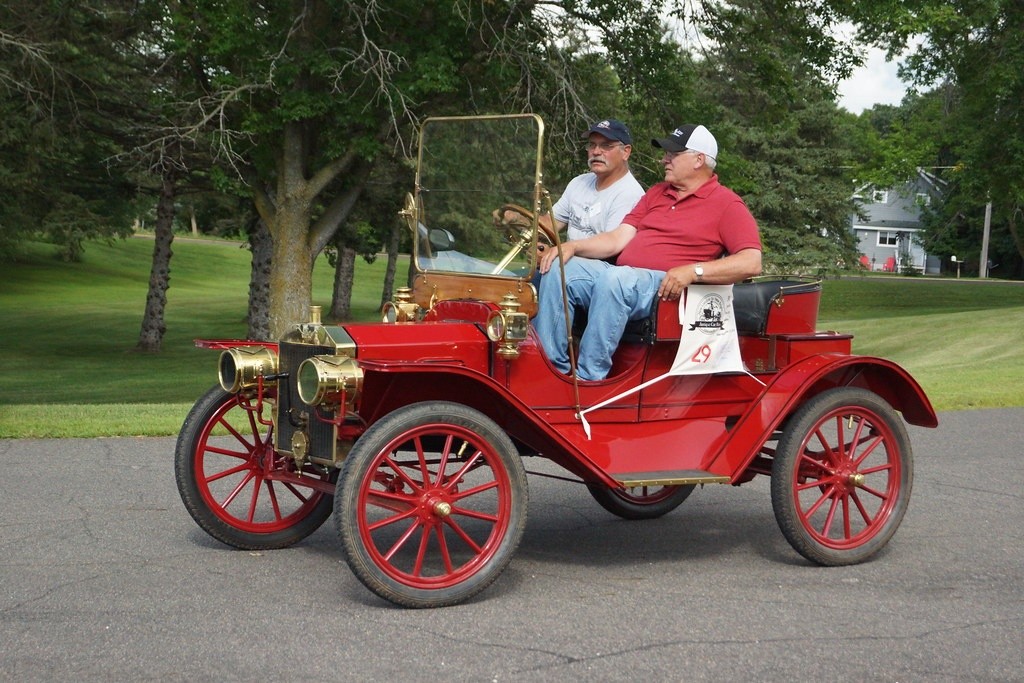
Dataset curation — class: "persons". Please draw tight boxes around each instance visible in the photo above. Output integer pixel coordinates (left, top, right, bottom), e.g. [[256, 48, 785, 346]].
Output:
[[535, 123, 762, 381], [492, 119, 646, 330]]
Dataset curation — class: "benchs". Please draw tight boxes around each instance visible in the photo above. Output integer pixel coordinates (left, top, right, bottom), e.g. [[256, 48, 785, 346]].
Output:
[[642, 270, 823, 343]]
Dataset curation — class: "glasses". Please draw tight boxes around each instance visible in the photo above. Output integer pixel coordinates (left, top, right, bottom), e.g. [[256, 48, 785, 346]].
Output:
[[663, 151, 695, 157], [584, 142, 622, 152]]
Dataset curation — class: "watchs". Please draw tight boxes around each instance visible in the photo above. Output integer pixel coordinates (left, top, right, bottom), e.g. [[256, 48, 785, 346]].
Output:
[[695, 263, 704, 282]]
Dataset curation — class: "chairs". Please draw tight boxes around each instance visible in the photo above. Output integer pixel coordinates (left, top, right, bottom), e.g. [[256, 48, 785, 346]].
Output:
[[883, 257, 895, 272], [860, 255, 871, 270]]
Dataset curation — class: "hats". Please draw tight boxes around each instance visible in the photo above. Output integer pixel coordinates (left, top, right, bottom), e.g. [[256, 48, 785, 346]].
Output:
[[581, 119, 632, 145], [651, 124, 718, 160]]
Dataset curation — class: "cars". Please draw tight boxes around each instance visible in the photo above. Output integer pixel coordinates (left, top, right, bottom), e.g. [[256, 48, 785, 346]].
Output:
[[174, 113, 938, 608]]
